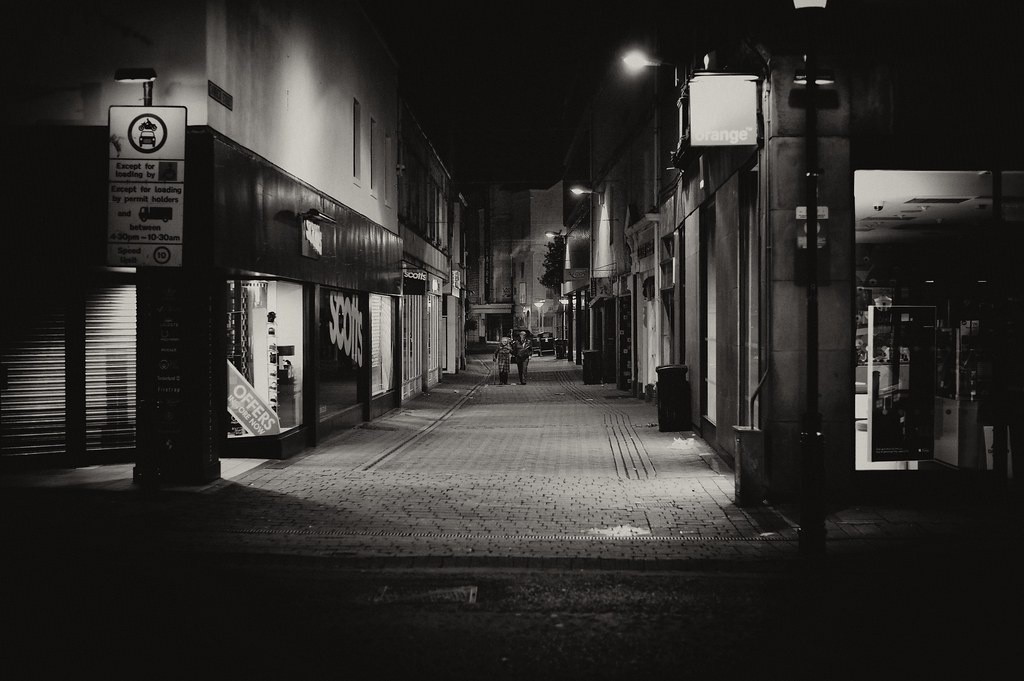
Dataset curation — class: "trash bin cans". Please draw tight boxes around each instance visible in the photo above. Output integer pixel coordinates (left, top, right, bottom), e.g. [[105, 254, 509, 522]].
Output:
[[554, 337, 567, 359], [656, 364, 691, 432], [582, 350, 602, 385]]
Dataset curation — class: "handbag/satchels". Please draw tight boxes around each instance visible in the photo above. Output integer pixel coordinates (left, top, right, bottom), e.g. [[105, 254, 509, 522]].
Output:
[[510, 354, 517, 364]]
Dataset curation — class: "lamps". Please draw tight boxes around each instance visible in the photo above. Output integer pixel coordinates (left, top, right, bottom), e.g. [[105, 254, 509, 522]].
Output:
[[113, 67, 157, 106], [546, 230, 560, 236], [570, 184, 604, 204]]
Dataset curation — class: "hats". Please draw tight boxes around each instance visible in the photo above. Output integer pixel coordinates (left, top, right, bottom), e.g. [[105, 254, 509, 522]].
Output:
[[520, 331, 527, 336]]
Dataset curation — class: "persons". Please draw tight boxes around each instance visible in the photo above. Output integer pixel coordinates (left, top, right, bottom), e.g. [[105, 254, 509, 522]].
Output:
[[493, 331, 532, 385]]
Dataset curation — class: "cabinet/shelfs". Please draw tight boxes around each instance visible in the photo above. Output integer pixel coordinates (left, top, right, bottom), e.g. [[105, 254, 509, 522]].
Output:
[[856, 285, 983, 470]]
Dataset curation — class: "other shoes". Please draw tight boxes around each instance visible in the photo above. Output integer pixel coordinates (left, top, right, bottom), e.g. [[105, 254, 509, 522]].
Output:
[[499, 381, 503, 385], [521, 379, 527, 385], [505, 382, 510, 385]]
